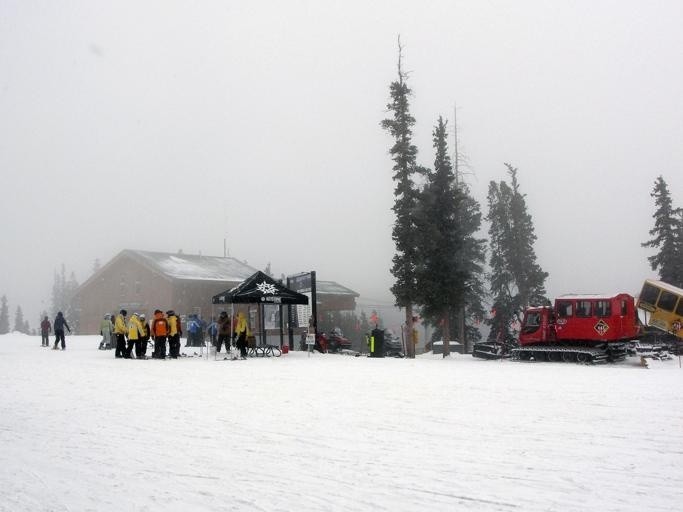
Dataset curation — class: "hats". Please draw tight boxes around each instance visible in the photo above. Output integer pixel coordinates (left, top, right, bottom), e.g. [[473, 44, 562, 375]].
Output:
[[119, 310, 175, 320]]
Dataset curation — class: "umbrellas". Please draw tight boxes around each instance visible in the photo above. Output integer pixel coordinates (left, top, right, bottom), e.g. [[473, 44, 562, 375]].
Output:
[[210, 267, 307, 342]]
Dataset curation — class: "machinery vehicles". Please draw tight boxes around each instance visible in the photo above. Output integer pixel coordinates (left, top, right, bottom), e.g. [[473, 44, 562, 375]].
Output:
[[511, 294, 640, 365]]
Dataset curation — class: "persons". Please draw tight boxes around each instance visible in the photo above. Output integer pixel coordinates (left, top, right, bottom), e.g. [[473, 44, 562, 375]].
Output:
[[51, 311, 70, 350], [98, 306, 252, 361], [40, 313, 50, 347]]
